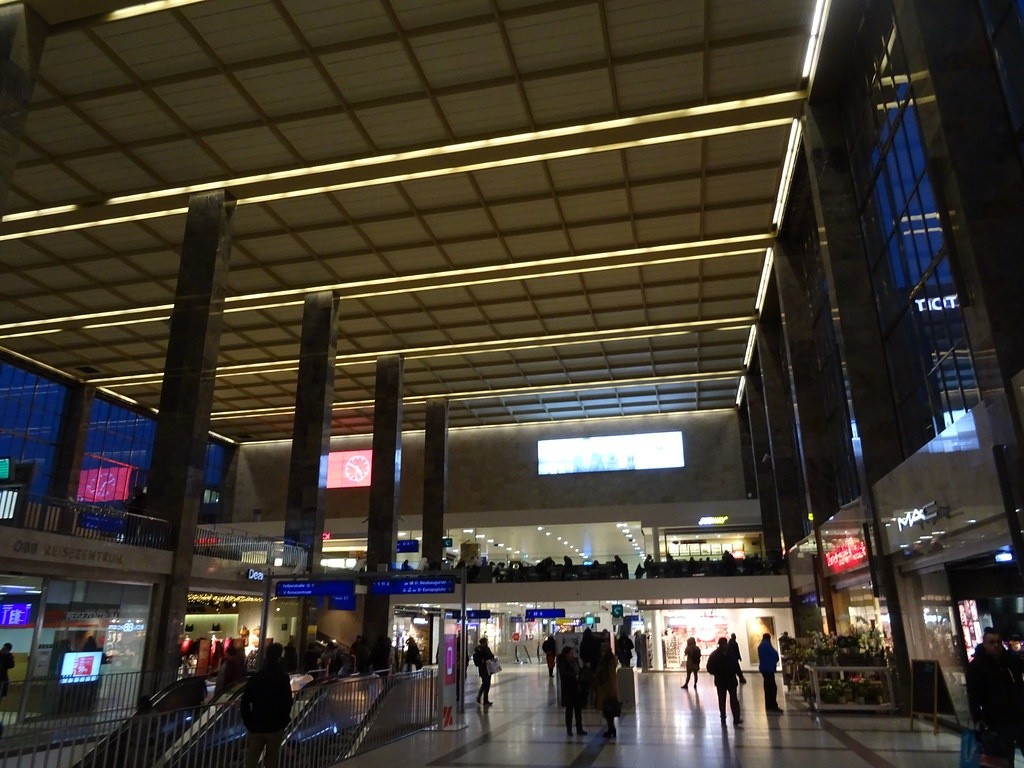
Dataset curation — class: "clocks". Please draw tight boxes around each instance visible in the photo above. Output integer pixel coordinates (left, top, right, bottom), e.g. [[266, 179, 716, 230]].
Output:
[[345, 455, 370, 480]]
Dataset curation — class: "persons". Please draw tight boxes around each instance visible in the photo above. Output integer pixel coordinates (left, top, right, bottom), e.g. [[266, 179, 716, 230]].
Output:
[[213, 635, 420, 768], [83, 636, 97, 652], [542, 628, 642, 737], [966, 627, 1024, 768], [564, 554, 623, 579], [475, 638, 495, 705], [404, 560, 409, 570], [645, 555, 653, 570], [681, 631, 790, 725], [0, 643, 15, 697]]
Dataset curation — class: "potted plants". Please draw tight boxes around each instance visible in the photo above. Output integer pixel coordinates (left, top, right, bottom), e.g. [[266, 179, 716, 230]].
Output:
[[788, 626, 895, 708]]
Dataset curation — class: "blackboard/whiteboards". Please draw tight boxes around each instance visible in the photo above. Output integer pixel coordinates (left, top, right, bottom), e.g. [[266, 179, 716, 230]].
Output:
[[910, 658, 938, 718]]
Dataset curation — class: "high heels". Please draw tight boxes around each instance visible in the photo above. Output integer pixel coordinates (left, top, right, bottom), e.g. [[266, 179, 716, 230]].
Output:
[[603, 727, 616, 738]]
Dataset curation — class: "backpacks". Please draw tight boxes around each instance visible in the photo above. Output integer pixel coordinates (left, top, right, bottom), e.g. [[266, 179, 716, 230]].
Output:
[[707, 649, 726, 674]]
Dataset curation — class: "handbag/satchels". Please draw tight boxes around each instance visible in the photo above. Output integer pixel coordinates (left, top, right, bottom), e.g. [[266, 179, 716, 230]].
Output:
[[486, 657, 502, 675], [959, 715, 980, 768]]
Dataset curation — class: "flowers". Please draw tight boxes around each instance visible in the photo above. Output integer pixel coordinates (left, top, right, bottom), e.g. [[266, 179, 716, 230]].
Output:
[[836, 626, 864, 637]]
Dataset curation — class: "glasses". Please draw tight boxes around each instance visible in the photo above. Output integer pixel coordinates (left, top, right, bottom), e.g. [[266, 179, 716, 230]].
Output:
[[985, 640, 1002, 644]]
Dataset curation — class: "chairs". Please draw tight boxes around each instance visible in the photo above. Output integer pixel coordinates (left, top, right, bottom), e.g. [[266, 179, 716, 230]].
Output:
[[76, 466, 132, 536]]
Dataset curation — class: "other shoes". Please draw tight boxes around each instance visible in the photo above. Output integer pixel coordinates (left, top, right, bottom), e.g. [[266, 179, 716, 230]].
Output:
[[484, 700, 493, 705], [577, 729, 587, 735], [721, 717, 726, 722], [767, 708, 783, 714], [681, 684, 687, 688], [734, 720, 743, 726], [567, 729, 573, 735], [477, 697, 481, 704]]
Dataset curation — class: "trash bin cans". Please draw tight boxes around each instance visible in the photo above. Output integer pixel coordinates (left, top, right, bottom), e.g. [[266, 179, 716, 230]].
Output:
[[616, 666, 639, 707]]
